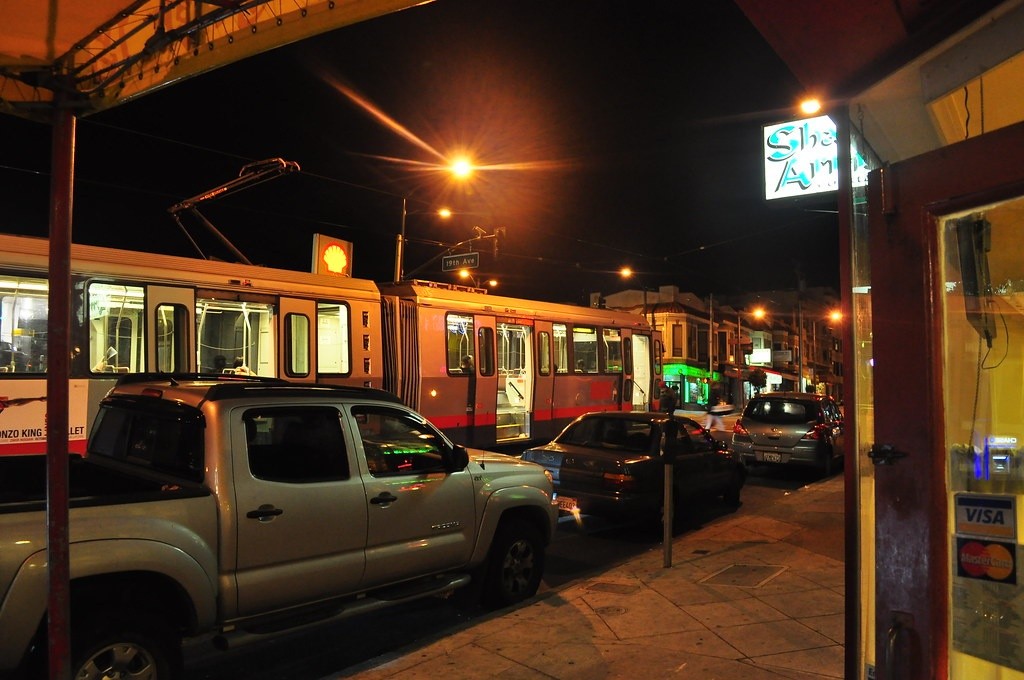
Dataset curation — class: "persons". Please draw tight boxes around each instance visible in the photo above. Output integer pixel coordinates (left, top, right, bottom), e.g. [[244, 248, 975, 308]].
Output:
[[458, 355, 475, 375], [705, 381, 724, 432], [229, 355, 257, 376], [577, 360, 588, 373], [208, 355, 230, 374], [666, 386, 679, 419]]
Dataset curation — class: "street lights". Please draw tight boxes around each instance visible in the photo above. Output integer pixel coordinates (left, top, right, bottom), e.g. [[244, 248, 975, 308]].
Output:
[[401, 159, 472, 281], [738, 310, 765, 410], [812, 312, 842, 392], [620, 267, 647, 320], [460, 271, 498, 290]]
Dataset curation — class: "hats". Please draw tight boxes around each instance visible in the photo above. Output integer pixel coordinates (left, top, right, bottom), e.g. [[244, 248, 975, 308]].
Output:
[[658, 381, 666, 387]]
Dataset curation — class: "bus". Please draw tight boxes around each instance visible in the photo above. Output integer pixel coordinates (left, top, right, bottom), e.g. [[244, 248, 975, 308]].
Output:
[[0, 234, 665, 509]]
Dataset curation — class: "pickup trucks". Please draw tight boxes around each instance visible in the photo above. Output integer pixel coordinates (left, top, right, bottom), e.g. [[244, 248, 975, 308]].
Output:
[[1, 371, 560, 680]]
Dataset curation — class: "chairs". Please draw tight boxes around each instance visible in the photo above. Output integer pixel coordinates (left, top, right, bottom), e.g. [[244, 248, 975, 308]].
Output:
[[517, 369, 526, 383], [498, 367, 508, 391], [629, 433, 650, 449]]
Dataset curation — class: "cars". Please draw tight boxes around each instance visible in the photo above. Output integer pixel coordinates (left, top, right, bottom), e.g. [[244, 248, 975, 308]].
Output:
[[731, 393, 844, 477], [520, 411, 748, 542]]
